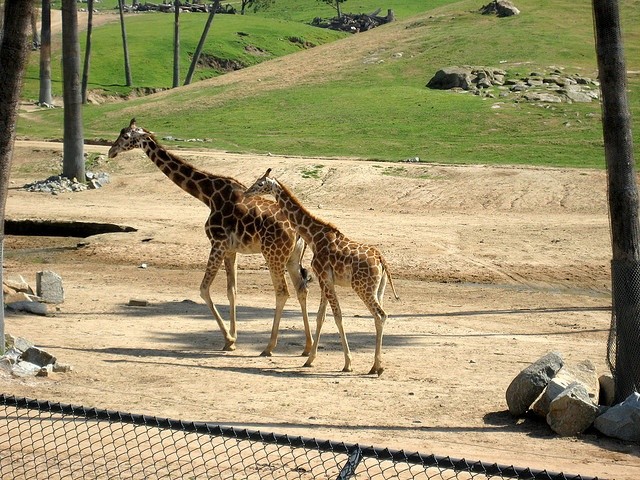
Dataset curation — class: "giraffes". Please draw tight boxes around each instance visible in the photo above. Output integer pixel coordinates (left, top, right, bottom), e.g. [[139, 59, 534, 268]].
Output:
[[108, 117, 315, 357], [241, 168, 399, 377]]
